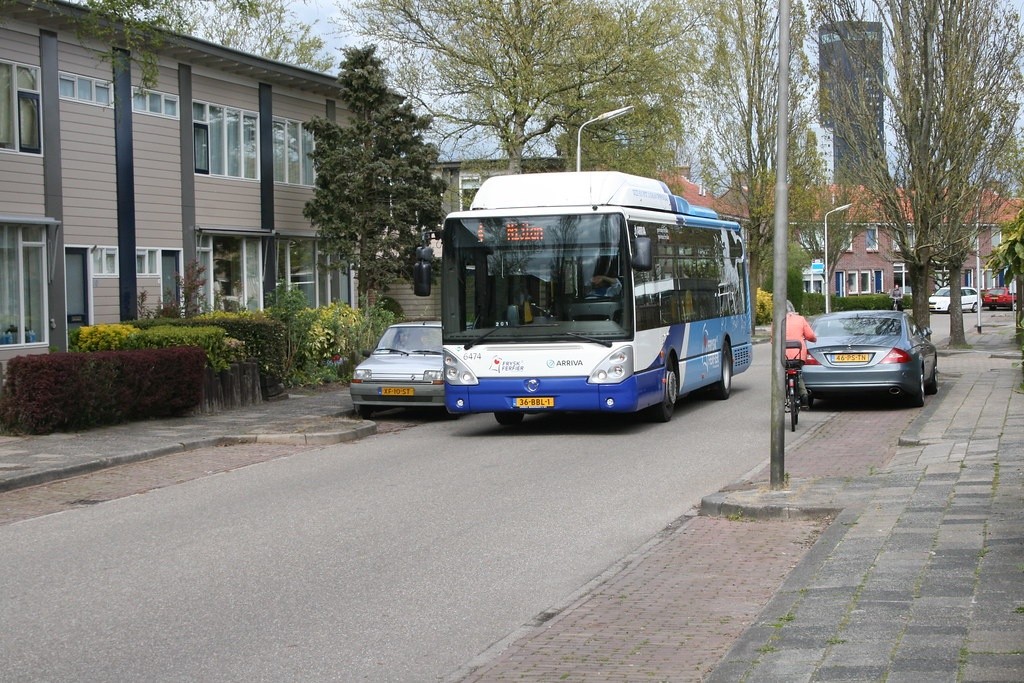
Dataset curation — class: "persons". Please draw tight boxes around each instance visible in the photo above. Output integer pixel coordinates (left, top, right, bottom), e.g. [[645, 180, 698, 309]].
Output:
[[768, 297, 817, 407], [585, 269, 622, 301], [891, 284, 904, 311]]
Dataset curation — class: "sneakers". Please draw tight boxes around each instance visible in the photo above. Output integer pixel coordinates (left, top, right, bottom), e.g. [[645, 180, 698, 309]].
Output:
[[802, 394, 808, 406]]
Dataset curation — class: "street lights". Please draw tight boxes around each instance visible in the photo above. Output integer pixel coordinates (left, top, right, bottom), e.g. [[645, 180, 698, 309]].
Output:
[[577, 104, 636, 172], [824, 203, 852, 315]]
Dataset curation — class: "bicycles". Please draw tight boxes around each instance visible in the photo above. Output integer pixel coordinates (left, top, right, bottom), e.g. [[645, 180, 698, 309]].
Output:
[[771, 336, 810, 431], [889, 296, 903, 311]]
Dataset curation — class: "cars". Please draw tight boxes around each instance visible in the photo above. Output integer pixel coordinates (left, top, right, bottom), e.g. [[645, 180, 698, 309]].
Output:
[[351, 321, 473, 420], [801, 309, 938, 408], [985, 288, 1018, 310], [928, 287, 982, 314]]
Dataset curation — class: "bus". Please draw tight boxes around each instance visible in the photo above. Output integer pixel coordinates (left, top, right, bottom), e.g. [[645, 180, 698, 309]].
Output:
[[413, 170, 754, 425]]
